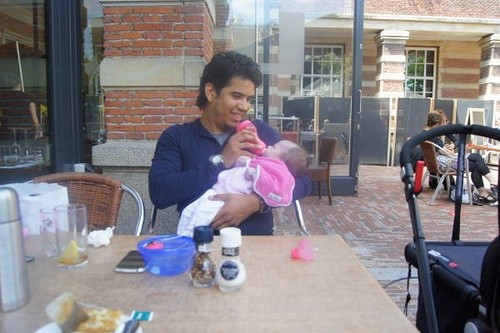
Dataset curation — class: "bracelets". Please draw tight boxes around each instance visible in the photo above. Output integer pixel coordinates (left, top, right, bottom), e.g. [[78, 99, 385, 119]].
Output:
[[258, 196, 264, 213]]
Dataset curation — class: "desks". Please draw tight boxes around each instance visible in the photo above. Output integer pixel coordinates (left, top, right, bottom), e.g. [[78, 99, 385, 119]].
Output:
[[300, 131, 324, 146], [0, 234, 420, 333]]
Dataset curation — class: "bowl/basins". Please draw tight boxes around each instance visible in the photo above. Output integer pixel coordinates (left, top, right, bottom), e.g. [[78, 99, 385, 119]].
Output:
[[136, 234, 196, 276]]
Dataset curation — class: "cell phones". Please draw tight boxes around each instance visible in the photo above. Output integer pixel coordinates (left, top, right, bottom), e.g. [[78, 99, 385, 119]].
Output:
[[115, 250, 145, 273]]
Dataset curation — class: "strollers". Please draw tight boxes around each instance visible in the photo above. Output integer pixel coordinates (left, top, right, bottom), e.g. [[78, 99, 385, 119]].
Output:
[[399, 123, 500, 333]]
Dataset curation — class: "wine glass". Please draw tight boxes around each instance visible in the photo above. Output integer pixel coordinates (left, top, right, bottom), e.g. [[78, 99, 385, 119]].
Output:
[[0, 125, 50, 166]]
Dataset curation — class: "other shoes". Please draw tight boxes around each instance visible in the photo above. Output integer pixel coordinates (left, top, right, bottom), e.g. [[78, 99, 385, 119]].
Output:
[[477, 194, 497, 203], [490, 183, 500, 193]]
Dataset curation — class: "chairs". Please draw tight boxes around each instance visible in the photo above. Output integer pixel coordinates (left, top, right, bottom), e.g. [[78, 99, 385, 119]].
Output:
[[304, 136, 338, 204], [24, 173, 144, 236], [147, 200, 310, 237], [397, 122, 500, 333]]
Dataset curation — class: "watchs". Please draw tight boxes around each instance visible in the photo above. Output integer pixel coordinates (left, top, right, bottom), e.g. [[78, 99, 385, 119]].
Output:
[[211, 154, 227, 171]]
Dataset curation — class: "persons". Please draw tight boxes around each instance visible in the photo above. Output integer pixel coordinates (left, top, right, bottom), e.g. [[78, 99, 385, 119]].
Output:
[[149, 50, 312, 236], [438, 109, 457, 150], [422, 110, 498, 207], [177, 140, 310, 236], [0, 71, 39, 138]]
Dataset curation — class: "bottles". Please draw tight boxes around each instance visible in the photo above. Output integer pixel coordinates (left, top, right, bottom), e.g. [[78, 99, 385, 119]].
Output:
[[215, 226, 247, 293], [188, 225, 216, 288]]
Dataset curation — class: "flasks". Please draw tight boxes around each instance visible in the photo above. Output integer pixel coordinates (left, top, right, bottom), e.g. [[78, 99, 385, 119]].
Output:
[[0, 186, 30, 312]]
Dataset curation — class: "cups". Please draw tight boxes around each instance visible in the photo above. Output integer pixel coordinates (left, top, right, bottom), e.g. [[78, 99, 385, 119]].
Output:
[[54, 203, 89, 270], [39, 205, 77, 262]]
